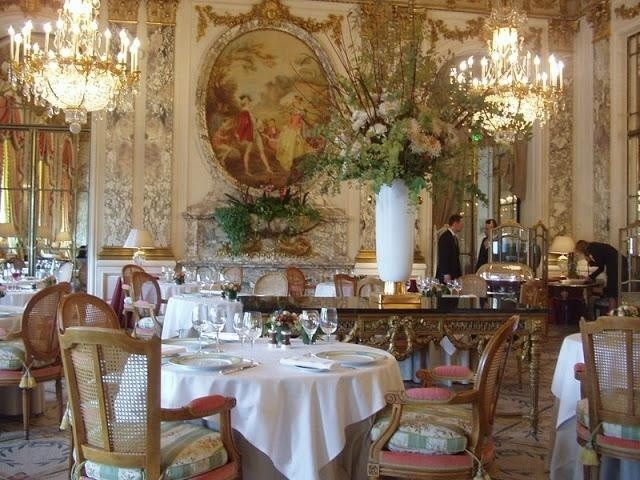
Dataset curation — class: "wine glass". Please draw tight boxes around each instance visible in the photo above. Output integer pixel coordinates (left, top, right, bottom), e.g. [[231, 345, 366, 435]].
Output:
[[419, 277, 463, 298], [302, 310, 320, 358], [320, 308, 339, 350], [191, 308, 264, 366], [4, 268, 24, 292], [155, 263, 216, 298]]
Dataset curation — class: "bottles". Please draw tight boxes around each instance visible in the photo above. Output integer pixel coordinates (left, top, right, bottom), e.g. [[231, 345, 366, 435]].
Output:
[[268, 330, 293, 349], [35, 260, 51, 279]]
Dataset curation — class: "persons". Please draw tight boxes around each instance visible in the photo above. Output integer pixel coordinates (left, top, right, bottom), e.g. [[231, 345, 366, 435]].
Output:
[[477, 219, 503, 271], [436, 214, 466, 283], [207, 92, 318, 179], [574, 238, 630, 313]]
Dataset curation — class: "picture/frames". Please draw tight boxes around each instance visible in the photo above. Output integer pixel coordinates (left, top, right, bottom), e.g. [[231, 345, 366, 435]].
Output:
[[194, 18, 341, 201]]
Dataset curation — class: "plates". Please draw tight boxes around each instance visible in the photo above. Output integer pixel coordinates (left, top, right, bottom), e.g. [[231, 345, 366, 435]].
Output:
[[314, 350, 387, 366], [162, 337, 218, 347], [170, 354, 242, 370], [295, 365, 327, 372]]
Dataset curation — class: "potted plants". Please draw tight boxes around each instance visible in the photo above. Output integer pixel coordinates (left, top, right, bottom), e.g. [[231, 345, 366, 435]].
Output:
[[213, 183, 320, 258]]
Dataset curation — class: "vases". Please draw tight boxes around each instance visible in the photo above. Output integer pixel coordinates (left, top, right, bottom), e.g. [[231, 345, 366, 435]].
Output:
[[370, 178, 425, 305]]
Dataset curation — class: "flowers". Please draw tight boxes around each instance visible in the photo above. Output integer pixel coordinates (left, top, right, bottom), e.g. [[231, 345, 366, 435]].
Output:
[[287, 0, 534, 223]]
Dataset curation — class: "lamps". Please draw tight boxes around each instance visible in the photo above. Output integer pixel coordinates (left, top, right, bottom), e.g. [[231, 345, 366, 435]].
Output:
[[448, 0, 566, 142], [7, 0, 141, 135], [548, 234, 577, 280], [121, 229, 155, 267]]
